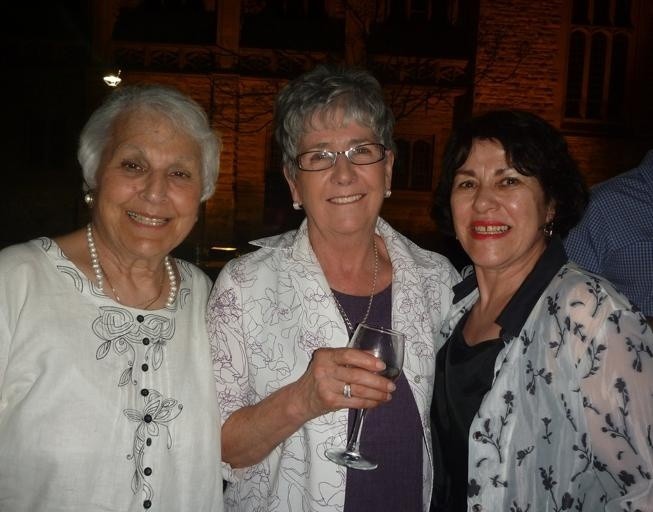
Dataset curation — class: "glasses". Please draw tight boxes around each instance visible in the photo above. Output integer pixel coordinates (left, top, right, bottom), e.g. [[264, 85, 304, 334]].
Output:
[[297, 143, 386, 171]]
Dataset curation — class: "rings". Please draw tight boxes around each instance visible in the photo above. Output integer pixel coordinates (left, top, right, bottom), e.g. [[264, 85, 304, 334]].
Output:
[[344, 382, 351, 399]]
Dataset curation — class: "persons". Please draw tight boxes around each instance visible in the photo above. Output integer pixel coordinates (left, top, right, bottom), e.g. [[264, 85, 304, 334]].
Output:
[[206, 64, 463, 511], [430, 108, 653, 512], [0, 83, 223, 512], [561, 149, 653, 325]]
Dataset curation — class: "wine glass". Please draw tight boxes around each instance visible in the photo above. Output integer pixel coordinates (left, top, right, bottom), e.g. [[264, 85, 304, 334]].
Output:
[[323, 323, 407, 473]]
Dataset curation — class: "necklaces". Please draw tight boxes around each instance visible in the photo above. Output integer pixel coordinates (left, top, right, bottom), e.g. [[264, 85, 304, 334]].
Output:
[[98, 261, 166, 310], [87, 221, 177, 310], [330, 234, 379, 334]]
